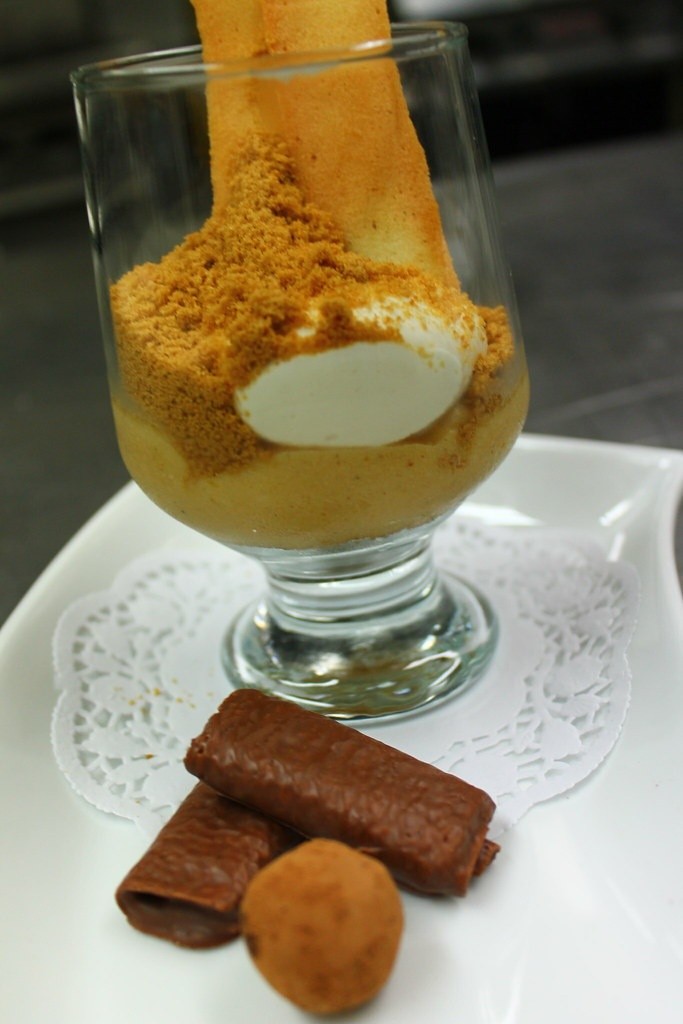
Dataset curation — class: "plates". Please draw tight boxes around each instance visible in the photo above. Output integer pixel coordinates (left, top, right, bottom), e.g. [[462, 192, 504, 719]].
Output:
[[0, 427, 682, 1024]]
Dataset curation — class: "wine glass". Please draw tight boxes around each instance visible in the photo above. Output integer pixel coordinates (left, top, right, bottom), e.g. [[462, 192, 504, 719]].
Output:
[[64, 21, 535, 728]]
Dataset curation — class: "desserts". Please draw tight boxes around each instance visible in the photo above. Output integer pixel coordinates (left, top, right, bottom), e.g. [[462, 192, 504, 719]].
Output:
[[112, 780, 302, 950], [183, 687, 502, 898]]
